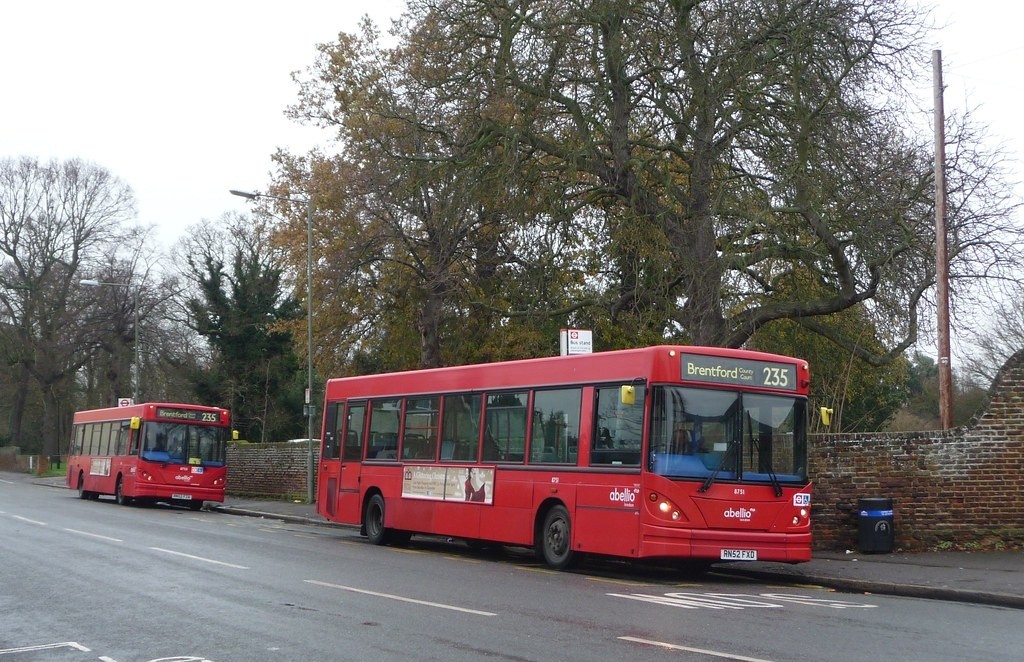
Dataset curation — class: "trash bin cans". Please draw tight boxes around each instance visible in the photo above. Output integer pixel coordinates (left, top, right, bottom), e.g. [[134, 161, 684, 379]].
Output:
[[857, 496, 894, 556]]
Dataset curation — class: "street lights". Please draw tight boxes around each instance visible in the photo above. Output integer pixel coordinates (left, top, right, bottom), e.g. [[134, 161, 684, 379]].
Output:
[[228, 189, 316, 506], [79, 278, 141, 405]]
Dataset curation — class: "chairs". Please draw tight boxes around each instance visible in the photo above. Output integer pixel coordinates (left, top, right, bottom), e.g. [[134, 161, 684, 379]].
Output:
[[336, 427, 578, 463]]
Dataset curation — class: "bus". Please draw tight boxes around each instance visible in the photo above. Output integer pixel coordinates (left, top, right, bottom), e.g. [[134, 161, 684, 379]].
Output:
[[314, 345, 835, 571], [65, 397, 232, 510]]
